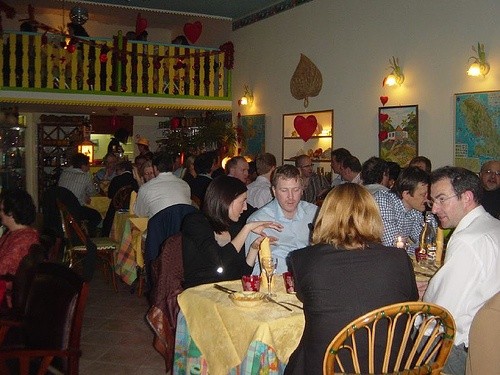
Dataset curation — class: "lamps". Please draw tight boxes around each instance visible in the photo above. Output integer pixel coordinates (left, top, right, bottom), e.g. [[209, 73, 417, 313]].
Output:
[[464, 41, 492, 79], [241, 79, 258, 106], [382, 54, 406, 89], [73, 135, 94, 167]]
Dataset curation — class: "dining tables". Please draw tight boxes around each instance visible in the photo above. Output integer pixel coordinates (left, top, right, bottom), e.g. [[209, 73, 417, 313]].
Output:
[[113, 208, 146, 296], [87, 191, 114, 219], [167, 271, 435, 375]]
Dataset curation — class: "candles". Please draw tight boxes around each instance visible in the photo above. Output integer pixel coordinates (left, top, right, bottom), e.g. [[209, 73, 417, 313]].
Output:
[[396, 235, 405, 248]]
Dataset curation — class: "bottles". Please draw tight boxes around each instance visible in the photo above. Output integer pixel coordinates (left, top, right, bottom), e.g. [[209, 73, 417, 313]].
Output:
[[420, 215, 437, 266]]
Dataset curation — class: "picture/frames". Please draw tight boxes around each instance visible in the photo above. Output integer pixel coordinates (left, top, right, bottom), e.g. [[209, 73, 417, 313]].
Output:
[[450, 89, 500, 176], [376, 104, 419, 168]]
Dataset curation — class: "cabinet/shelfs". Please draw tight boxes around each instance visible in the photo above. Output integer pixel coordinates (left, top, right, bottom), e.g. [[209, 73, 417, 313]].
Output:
[[281, 109, 335, 180]]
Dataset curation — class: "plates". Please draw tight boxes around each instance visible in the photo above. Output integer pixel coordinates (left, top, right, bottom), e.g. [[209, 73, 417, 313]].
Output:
[[230, 290, 266, 308]]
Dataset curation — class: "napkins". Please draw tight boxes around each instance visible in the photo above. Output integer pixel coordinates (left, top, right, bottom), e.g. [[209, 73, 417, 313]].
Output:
[[258, 236, 277, 289]]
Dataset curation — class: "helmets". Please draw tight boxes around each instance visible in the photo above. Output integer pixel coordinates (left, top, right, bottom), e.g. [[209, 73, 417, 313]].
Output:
[[135, 137, 150, 146]]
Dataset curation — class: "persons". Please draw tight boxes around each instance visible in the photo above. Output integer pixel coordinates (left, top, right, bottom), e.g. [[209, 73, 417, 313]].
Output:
[[477, 161, 500, 219], [373, 168, 429, 258], [413, 165, 500, 375], [182, 176, 284, 290], [282, 181, 419, 375], [244, 164, 318, 276], [4, 20, 190, 87], [0, 139, 432, 342]]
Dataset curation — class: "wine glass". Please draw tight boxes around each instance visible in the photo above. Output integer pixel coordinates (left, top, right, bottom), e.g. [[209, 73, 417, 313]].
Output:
[[262, 257, 278, 299]]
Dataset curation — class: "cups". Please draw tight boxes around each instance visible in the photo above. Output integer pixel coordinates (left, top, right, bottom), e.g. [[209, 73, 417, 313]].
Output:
[[241, 274, 261, 293], [415, 247, 427, 263], [283, 271, 295, 293]]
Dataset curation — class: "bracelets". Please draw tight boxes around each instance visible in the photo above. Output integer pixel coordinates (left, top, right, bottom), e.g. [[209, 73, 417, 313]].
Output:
[[250, 242, 260, 249]]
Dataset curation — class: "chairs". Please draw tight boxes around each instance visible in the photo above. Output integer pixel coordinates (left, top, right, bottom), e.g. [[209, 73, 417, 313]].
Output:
[[321, 300, 460, 375], [55, 196, 121, 294], [148, 232, 184, 366], [136, 202, 199, 296], [41, 188, 91, 242], [0, 266, 92, 375]]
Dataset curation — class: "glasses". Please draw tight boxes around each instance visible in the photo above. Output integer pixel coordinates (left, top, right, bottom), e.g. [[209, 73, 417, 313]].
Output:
[[299, 164, 314, 169], [426, 192, 463, 208], [481, 170, 500, 176]]
